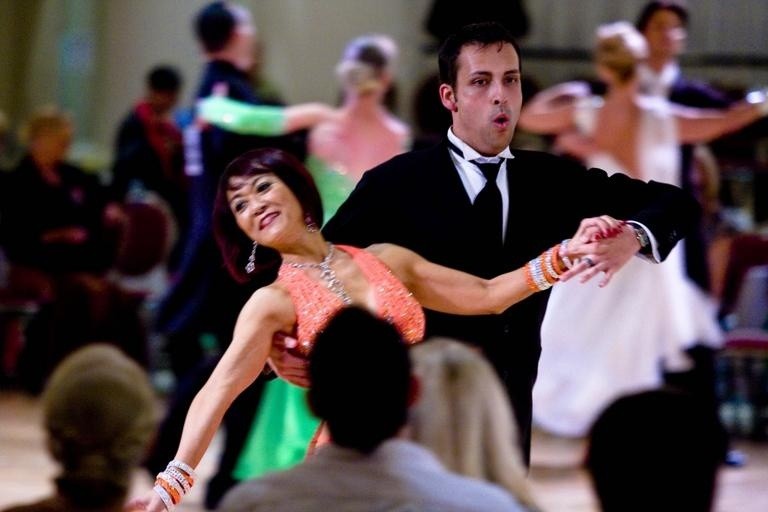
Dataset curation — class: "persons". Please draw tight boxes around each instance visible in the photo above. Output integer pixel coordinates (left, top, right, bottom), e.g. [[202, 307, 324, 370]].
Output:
[[213, 305, 538, 511], [520, 19, 767, 440], [1, 64, 767, 398], [194, 32, 409, 485], [145, 2, 279, 511], [143, 145, 629, 511], [2, 340, 184, 512], [543, 2, 748, 463], [586, 384, 719, 512], [315, 19, 701, 463]]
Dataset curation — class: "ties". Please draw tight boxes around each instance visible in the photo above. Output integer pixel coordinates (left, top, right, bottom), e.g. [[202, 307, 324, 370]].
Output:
[[446, 140, 506, 246]]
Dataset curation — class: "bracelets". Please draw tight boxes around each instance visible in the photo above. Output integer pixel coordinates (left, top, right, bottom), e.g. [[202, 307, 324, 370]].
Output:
[[152, 459, 198, 511]]
